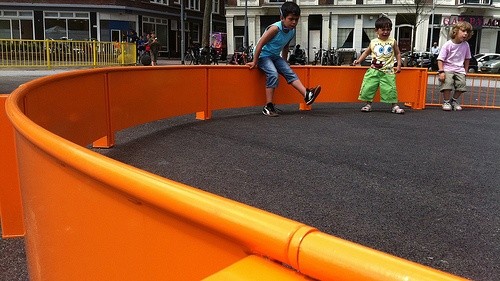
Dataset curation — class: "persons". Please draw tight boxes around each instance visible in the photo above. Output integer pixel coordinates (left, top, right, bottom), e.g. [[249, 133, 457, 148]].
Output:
[[353, 17, 405, 114], [431, 20, 473, 110], [122, 30, 159, 67], [245, 0, 321, 116], [114, 39, 121, 55]]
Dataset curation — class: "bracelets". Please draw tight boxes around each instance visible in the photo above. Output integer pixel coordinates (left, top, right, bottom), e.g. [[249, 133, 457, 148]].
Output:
[[438, 69, 445, 73]]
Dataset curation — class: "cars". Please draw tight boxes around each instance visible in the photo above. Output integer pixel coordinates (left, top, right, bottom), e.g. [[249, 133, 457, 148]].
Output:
[[349, 47, 500, 74]]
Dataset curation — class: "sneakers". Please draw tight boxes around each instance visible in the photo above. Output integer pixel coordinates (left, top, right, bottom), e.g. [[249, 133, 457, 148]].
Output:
[[451, 102, 463, 111], [442, 101, 452, 111], [361, 105, 372, 112], [392, 106, 405, 114], [304, 83, 321, 105], [262, 105, 279, 117]]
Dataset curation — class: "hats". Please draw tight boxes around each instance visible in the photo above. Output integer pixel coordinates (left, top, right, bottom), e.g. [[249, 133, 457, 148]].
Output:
[[141, 55, 151, 65], [434, 42, 437, 45]]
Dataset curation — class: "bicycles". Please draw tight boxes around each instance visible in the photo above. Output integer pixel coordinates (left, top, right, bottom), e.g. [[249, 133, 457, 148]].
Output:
[[226, 43, 253, 65], [137, 43, 151, 66], [183, 40, 219, 65], [287, 44, 345, 66]]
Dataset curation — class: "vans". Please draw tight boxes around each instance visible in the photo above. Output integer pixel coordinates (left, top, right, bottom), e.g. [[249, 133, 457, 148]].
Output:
[[209, 31, 227, 62]]
